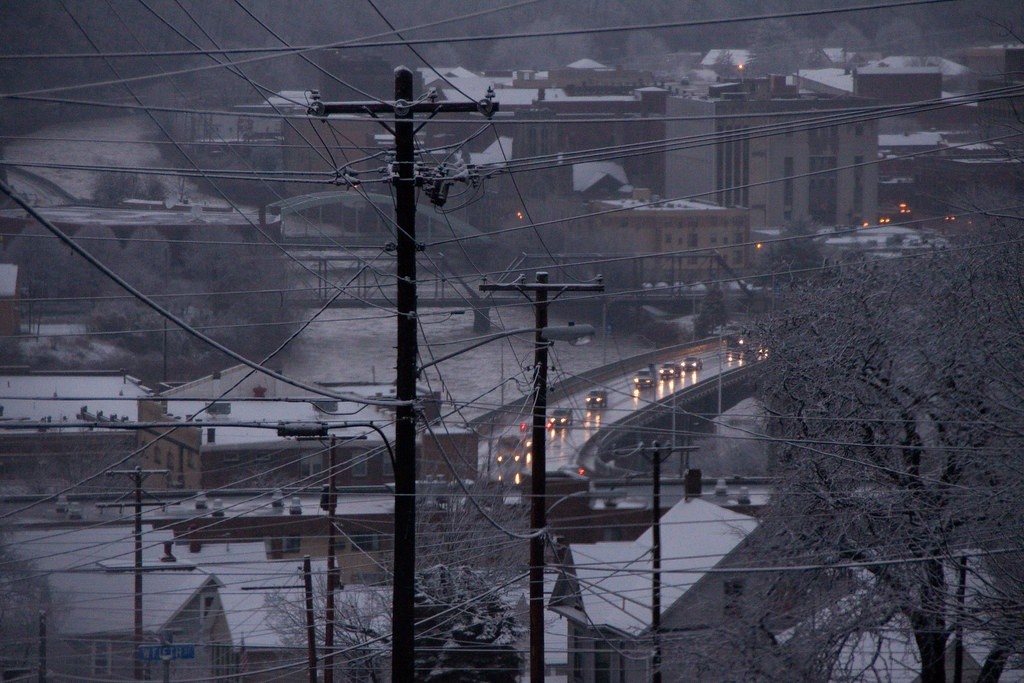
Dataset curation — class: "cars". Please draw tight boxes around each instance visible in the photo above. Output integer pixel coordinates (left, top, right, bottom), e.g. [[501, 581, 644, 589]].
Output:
[[584, 390, 608, 410], [524, 434, 533, 452], [548, 406, 573, 428], [495, 436, 526, 469], [682, 356, 703, 371], [633, 370, 654, 388], [660, 362, 681, 379]]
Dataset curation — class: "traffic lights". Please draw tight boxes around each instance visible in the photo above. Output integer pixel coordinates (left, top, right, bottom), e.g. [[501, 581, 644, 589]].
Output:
[[520, 423, 529, 433]]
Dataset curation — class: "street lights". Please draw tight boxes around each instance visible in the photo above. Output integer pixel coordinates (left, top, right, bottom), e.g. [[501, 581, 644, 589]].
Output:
[[390, 322, 595, 683]]
